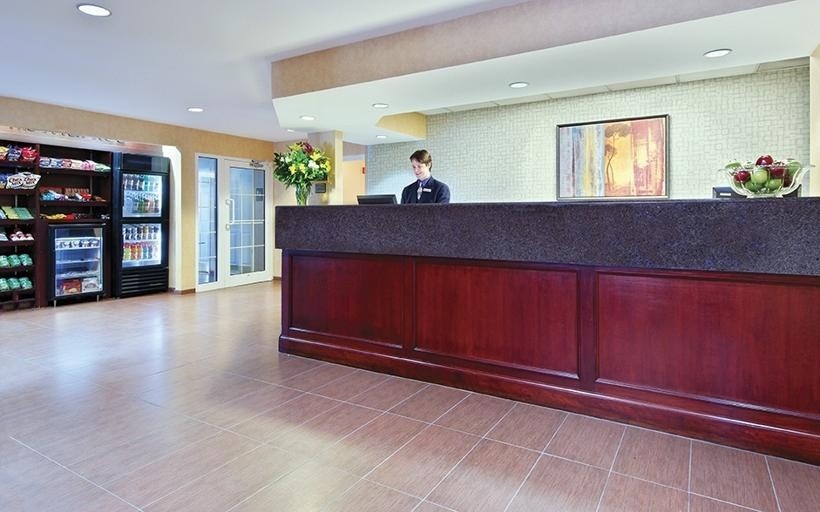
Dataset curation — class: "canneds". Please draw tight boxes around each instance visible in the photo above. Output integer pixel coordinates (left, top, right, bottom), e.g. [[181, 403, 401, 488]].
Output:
[[122, 223, 159, 241]]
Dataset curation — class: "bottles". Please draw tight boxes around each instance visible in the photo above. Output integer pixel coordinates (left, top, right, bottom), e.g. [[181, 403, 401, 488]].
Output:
[[122, 224, 161, 259], [123, 174, 162, 216]]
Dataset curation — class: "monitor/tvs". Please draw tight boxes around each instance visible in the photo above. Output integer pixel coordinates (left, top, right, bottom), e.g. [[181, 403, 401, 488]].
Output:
[[357, 194, 397, 204]]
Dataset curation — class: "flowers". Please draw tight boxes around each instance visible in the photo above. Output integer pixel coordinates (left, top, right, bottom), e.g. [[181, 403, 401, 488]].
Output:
[[273, 141, 331, 199]]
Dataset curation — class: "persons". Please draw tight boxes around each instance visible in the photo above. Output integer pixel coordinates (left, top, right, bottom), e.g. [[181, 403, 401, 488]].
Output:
[[399, 150, 450, 204]]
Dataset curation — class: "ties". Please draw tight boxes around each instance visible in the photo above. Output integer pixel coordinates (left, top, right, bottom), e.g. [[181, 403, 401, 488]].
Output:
[[416, 182, 423, 200]]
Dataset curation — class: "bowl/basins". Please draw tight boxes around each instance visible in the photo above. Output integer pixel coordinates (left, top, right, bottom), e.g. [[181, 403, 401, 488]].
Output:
[[716, 163, 815, 200]]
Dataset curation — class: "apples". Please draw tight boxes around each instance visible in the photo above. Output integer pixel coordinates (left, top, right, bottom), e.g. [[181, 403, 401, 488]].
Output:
[[724, 154, 801, 193]]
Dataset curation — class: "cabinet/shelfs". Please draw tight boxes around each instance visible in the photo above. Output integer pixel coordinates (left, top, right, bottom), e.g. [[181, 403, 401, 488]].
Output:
[[0, 138, 112, 310]]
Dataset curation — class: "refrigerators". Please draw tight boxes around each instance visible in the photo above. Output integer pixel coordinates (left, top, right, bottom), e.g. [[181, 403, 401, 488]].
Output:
[[111, 152, 170, 296]]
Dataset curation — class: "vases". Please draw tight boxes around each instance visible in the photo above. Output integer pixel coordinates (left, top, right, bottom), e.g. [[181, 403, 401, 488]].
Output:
[[296, 184, 308, 206]]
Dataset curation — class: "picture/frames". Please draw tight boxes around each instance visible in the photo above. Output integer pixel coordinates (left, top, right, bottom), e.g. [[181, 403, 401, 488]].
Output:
[[554, 113, 670, 200]]
